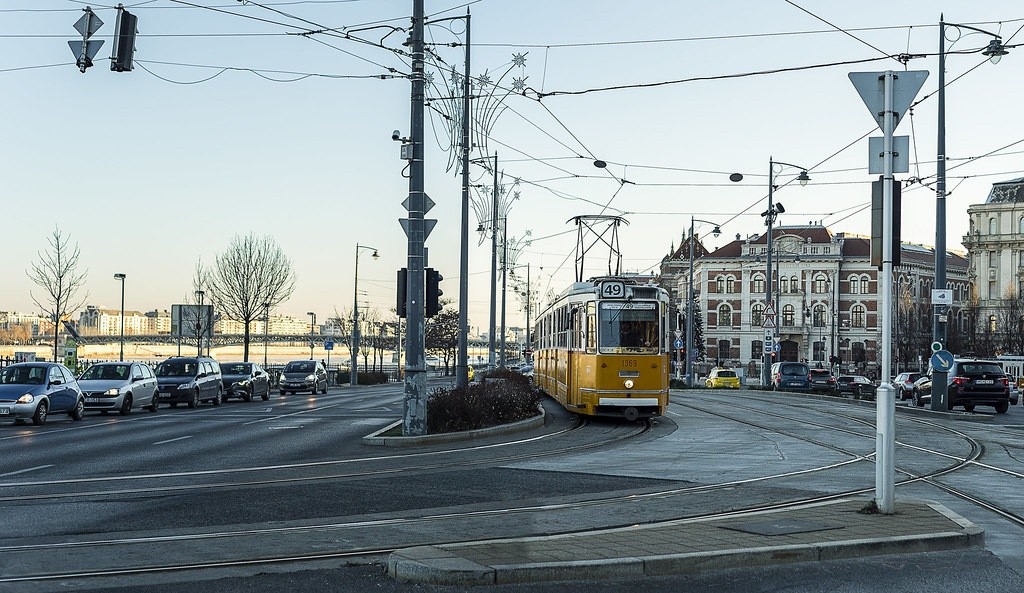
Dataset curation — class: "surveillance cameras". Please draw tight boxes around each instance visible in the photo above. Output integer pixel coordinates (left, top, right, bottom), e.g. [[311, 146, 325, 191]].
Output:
[[392, 130, 400, 141]]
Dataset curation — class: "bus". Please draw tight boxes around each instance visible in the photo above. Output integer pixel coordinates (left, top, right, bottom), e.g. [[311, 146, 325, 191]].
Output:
[[531, 214, 682, 421]]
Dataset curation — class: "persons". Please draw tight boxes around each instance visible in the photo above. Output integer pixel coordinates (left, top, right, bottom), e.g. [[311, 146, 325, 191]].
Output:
[[185, 364, 195, 375], [321, 359, 326, 369]]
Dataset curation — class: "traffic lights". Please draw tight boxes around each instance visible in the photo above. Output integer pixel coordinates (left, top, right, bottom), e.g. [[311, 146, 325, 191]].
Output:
[[424, 267, 443, 319], [110, 10, 140, 72]]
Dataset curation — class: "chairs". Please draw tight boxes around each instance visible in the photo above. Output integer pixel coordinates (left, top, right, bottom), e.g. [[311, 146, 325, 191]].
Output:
[[17, 372, 28, 384], [101, 370, 112, 379], [123, 370, 134, 379], [30, 377, 41, 385]]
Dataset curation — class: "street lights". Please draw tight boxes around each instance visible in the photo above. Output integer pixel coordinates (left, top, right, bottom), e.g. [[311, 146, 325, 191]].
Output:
[[476, 212, 509, 368], [754, 245, 801, 362], [509, 263, 532, 363], [845, 338, 851, 376], [822, 271, 844, 376], [459, 150, 500, 367], [113, 273, 127, 362], [307, 311, 314, 360], [763, 155, 812, 387], [195, 289, 205, 355], [351, 241, 381, 385], [683, 215, 723, 387], [262, 303, 271, 370], [863, 339, 868, 377], [928, 11, 1011, 408]]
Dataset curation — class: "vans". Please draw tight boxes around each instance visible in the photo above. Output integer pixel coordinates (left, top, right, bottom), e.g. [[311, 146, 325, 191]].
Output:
[[771, 361, 813, 393]]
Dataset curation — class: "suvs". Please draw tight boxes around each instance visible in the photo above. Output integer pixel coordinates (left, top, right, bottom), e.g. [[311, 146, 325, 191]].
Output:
[[810, 368, 839, 394], [278, 359, 329, 396], [911, 353, 1010, 414], [153, 355, 224, 409]]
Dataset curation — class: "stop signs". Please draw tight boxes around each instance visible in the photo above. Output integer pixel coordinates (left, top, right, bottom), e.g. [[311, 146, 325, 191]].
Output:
[[526, 350, 532, 355]]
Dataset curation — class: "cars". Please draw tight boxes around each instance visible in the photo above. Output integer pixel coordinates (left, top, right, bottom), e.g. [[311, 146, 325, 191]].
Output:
[[76, 361, 160, 415], [468, 360, 534, 385], [891, 371, 922, 401], [0, 361, 86, 426], [705, 367, 741, 390], [219, 362, 272, 403], [1005, 372, 1019, 405], [837, 374, 878, 398]]
